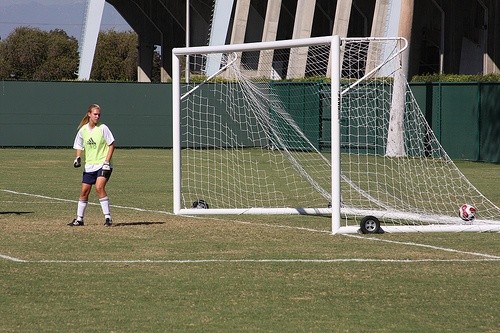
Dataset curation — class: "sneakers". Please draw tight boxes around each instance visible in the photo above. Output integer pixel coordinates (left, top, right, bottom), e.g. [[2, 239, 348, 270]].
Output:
[[67, 219, 84, 226], [104, 218, 112, 227]]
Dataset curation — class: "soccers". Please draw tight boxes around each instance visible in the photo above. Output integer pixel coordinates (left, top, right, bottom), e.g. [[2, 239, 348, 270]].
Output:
[[459, 204, 476, 221]]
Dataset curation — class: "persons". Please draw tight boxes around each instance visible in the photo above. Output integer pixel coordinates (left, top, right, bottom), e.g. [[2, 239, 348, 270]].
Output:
[[67, 104, 115, 227]]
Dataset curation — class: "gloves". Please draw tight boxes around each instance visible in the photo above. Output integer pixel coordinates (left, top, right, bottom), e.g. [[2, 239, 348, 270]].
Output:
[[102, 163, 111, 178], [73, 156, 81, 168]]
